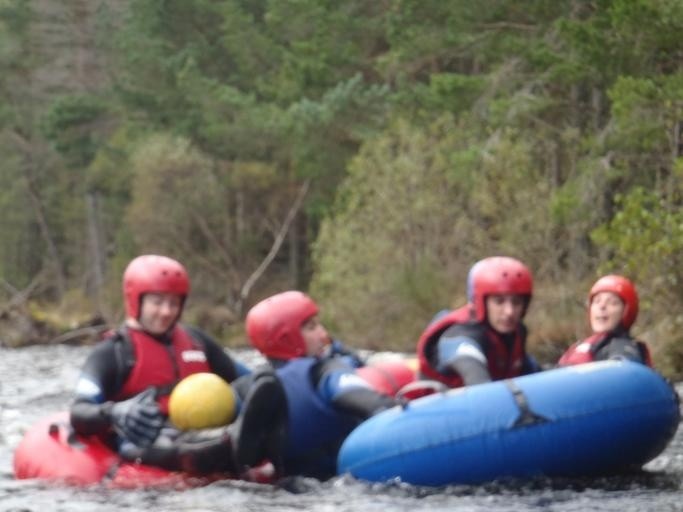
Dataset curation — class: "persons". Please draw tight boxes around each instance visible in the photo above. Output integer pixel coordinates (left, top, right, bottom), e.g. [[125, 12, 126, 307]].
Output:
[[417, 255, 534, 386], [245, 291, 414, 480], [67, 253, 283, 479], [553, 275, 650, 369]]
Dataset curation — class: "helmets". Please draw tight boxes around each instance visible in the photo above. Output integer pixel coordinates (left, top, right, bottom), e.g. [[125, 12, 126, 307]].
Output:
[[122, 253, 189, 318], [588, 274, 639, 328], [467, 255, 533, 322], [245, 290, 320, 359]]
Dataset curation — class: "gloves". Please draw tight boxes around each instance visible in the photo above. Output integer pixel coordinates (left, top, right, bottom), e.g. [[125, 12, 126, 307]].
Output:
[[107, 385, 163, 448]]
